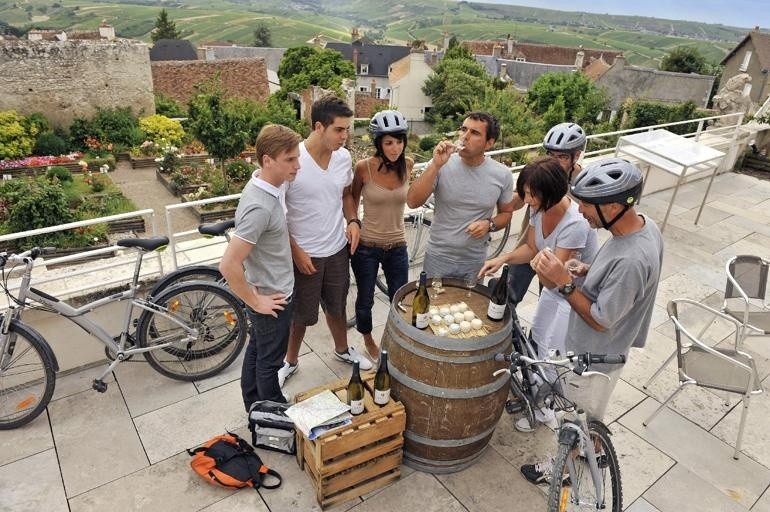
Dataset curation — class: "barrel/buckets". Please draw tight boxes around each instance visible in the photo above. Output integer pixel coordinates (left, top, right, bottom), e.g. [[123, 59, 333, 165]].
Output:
[[378, 276, 514, 475]]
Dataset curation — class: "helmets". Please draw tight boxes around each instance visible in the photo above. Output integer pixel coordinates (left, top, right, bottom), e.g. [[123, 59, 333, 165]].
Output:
[[570, 158, 643, 204], [543, 123, 586, 152], [368, 110, 408, 133]]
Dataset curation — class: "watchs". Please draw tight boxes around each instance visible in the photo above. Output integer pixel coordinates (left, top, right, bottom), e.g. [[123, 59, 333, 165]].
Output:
[[559, 282, 576, 296], [348, 219, 362, 229], [487, 218, 496, 232]]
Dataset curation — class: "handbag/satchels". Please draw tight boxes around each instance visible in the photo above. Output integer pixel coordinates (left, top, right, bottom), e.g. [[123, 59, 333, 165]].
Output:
[[247, 400, 296, 455]]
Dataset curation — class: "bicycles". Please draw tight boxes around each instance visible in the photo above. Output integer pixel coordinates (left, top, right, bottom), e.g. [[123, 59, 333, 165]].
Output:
[[147, 219, 360, 358], [376, 173, 511, 300], [487, 275, 624, 511], [0, 236, 247, 429]]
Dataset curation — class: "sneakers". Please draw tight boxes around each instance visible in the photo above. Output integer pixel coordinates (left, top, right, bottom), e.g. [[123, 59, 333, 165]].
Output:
[[521, 464, 571, 486], [365, 344, 378, 363], [515, 416, 539, 432], [578, 451, 610, 468], [334, 348, 372, 370], [277, 361, 299, 388], [283, 394, 290, 401]]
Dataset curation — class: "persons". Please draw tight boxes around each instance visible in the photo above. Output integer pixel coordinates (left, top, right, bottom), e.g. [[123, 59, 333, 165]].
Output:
[[506, 122, 586, 373], [520, 157, 664, 488], [278, 96, 373, 390], [352, 109, 415, 364], [218, 122, 302, 412], [477, 156, 599, 433], [407, 112, 514, 286]]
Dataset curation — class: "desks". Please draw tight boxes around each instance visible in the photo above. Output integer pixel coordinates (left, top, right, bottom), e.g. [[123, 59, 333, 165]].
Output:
[[613, 129, 727, 234]]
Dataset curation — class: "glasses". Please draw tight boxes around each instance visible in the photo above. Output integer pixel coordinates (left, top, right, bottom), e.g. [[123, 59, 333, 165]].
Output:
[[546, 152, 569, 160]]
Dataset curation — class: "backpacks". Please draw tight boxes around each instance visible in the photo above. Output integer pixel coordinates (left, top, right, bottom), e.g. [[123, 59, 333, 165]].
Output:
[[186, 432, 282, 490]]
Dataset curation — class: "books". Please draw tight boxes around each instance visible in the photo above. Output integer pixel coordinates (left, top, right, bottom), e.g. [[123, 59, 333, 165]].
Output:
[[284, 389, 354, 441]]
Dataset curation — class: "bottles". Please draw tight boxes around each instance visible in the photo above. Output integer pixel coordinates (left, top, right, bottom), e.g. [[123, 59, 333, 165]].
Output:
[[487, 265, 509, 322], [412, 271, 429, 330], [373, 351, 391, 406], [348, 360, 364, 415]]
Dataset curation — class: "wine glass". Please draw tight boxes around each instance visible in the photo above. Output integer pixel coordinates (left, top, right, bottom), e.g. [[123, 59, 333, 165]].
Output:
[[431, 278, 442, 300], [451, 140, 465, 153], [568, 251, 582, 279], [465, 274, 477, 297]]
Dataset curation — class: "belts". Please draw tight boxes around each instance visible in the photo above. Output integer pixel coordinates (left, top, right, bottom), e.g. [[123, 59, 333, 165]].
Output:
[[360, 239, 407, 249]]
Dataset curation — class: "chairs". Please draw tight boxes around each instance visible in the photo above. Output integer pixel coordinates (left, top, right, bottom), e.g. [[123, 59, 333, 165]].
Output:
[[642, 298, 763, 460], [693, 255, 770, 350]]
